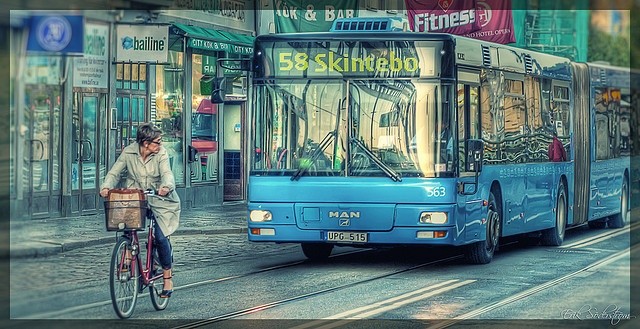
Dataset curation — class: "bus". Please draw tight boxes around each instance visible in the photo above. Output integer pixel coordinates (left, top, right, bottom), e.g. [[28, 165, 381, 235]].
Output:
[[212, 16, 640, 265]]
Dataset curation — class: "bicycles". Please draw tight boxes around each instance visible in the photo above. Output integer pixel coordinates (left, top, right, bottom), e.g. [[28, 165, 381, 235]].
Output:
[[98, 189, 174, 319]]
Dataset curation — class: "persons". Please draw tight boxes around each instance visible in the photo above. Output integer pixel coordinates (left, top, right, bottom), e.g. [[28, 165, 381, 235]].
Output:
[[99, 121, 181, 298], [619, 117, 630, 149], [549, 132, 566, 162], [409, 116, 453, 160]]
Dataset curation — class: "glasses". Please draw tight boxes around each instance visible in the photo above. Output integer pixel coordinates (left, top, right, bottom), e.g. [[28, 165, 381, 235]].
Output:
[[144, 137, 162, 144]]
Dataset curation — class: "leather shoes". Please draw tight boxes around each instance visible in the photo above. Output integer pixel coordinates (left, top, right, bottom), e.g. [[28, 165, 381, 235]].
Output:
[[161, 275, 173, 297], [118, 257, 132, 270]]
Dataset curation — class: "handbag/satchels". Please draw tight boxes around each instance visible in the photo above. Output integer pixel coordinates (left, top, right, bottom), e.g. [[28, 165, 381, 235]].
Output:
[[108, 188, 145, 229]]
